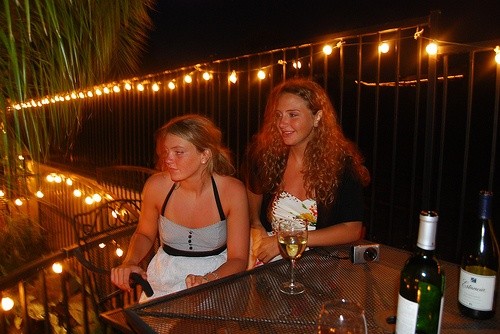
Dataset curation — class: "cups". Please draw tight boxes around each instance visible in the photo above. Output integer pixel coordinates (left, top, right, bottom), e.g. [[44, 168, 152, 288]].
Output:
[[315, 299, 367, 334]]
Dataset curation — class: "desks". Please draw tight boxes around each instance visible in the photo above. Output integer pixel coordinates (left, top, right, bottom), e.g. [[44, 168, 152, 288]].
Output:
[[101, 240, 500, 334]]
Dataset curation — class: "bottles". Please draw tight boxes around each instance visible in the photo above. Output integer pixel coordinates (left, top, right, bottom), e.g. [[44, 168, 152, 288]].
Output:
[[458, 190, 500, 321], [395, 209, 445, 334]]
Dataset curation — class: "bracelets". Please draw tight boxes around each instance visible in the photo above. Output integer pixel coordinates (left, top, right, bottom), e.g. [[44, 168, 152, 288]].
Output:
[[212, 272, 219, 279], [203, 275, 211, 282]]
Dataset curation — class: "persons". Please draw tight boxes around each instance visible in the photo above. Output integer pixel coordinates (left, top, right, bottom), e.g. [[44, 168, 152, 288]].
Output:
[[252, 80, 371, 267], [110, 116, 248, 303]]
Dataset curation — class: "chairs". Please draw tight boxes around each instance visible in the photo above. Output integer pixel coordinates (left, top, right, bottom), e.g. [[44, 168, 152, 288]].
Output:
[[73, 199, 154, 315]]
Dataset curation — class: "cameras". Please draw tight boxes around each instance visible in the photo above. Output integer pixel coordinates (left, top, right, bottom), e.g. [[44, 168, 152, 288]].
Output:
[[351, 244, 379, 264]]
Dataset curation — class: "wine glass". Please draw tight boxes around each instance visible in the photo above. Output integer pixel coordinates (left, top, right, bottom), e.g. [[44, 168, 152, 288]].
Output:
[[278, 218, 309, 296]]
